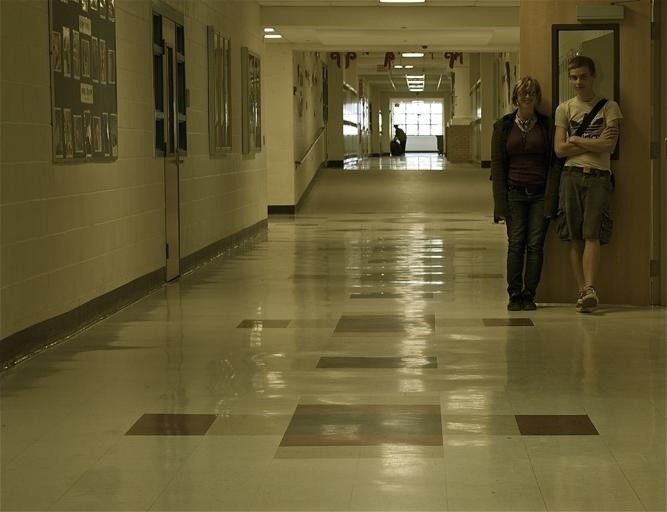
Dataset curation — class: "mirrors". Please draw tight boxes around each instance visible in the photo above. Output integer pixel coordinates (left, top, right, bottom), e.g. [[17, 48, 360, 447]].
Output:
[[551, 22, 622, 162]]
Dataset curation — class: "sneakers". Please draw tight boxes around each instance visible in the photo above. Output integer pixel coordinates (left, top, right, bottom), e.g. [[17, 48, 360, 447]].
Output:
[[507, 295, 537, 311], [576, 286, 599, 312]]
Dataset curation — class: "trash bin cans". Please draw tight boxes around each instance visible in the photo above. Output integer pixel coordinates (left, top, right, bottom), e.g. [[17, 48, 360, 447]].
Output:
[[436, 135, 443, 155]]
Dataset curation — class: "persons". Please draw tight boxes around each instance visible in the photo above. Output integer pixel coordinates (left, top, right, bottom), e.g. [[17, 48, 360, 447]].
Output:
[[393, 125, 406, 153], [489, 75, 564, 311], [554, 54, 624, 313]]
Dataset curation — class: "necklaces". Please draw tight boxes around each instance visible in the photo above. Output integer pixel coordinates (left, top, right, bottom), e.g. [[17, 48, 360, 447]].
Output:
[[516, 113, 533, 126]]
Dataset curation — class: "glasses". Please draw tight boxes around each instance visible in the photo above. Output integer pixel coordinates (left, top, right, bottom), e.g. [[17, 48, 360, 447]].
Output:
[[518, 90, 539, 97]]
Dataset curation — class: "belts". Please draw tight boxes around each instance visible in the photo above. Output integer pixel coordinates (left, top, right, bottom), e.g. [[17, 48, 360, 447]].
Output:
[[562, 165, 609, 177], [507, 184, 544, 195]]
